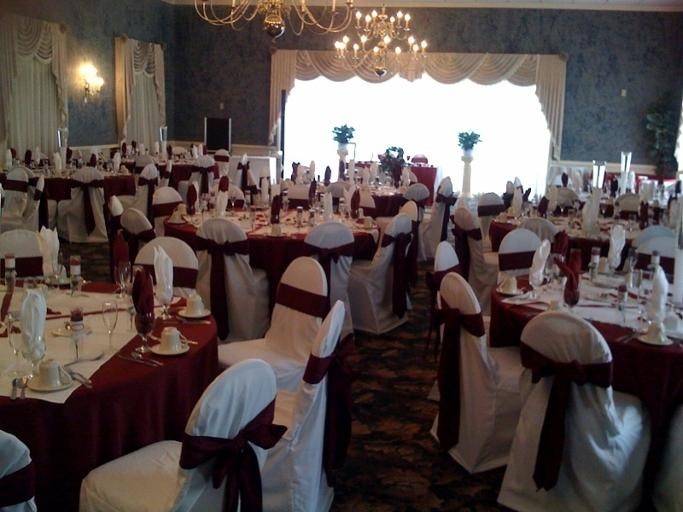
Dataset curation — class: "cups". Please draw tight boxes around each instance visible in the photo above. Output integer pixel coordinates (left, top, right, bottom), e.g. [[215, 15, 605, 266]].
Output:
[[39, 359, 71, 387], [646, 321, 667, 343], [187, 297, 204, 315], [160, 327, 187, 352]]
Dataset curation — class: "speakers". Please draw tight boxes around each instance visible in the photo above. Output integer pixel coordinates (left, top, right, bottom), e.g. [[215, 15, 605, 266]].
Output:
[[203, 117, 232, 153]]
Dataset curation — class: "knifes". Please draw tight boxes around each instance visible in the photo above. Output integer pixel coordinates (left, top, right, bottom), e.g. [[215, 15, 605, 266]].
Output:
[[12, 378, 17, 399]]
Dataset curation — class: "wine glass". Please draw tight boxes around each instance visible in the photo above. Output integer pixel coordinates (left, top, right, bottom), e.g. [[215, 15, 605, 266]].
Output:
[[4, 254, 174, 378]]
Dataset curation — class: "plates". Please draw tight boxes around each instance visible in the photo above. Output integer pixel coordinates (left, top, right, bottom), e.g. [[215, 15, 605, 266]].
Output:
[[26, 376, 72, 393], [637, 334, 674, 346], [149, 342, 190, 357], [177, 308, 211, 318]]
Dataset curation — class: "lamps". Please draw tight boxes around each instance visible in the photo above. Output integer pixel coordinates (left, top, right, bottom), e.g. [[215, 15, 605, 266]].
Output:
[[194, 0, 355, 42], [334, 0, 428, 76], [81, 65, 103, 102]]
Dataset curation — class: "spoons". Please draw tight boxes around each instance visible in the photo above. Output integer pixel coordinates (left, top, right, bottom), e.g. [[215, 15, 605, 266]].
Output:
[[18, 377, 27, 399]]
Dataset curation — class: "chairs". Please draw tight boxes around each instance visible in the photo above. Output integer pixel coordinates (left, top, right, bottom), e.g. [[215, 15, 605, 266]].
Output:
[[259, 299, 345, 512], [431, 271, 527, 472], [451, 176, 683, 276], [497, 309, 652, 511], [1, 140, 430, 345], [79, 359, 287, 512], [213, 255, 329, 390], [418, 177, 453, 260], [430, 242, 461, 339]]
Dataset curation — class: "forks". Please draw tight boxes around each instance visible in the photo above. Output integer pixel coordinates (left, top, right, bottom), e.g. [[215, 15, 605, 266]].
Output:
[[66, 367, 93, 384]]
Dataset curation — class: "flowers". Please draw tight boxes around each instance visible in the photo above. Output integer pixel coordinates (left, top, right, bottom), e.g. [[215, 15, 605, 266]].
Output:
[[456, 133, 482, 150], [332, 124, 355, 143]]
